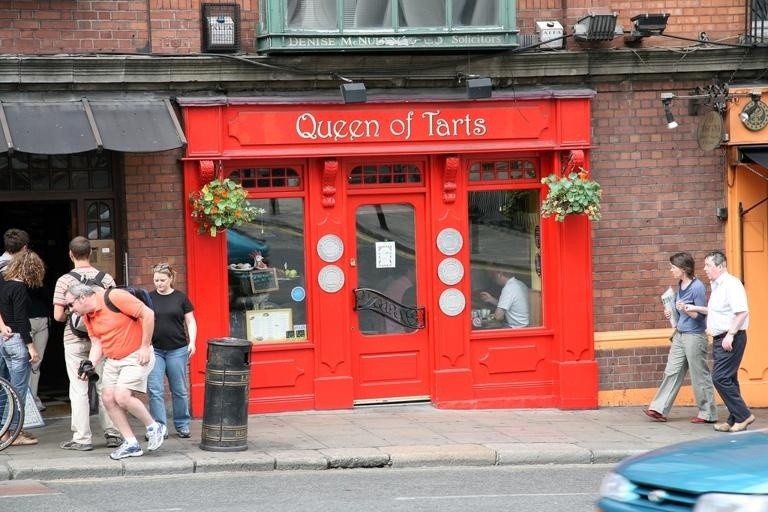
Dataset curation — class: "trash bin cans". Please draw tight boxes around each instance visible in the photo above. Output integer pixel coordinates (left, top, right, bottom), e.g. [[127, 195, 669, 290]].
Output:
[[198, 338, 254, 452]]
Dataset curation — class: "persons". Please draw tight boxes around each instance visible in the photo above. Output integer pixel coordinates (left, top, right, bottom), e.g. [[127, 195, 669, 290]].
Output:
[[144, 263, 196, 441], [684, 251, 756, 432], [1, 250, 45, 446], [642, 252, 719, 424], [53, 236, 125, 450], [480, 259, 530, 329], [1, 229, 49, 412], [63, 283, 168, 460]]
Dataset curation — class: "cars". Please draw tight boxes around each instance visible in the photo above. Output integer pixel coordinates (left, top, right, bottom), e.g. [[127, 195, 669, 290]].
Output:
[[226, 228, 271, 307], [592, 428, 767, 512]]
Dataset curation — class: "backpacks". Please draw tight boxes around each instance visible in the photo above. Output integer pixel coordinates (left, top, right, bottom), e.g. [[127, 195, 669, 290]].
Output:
[[67, 271, 106, 339], [87, 285, 153, 321]]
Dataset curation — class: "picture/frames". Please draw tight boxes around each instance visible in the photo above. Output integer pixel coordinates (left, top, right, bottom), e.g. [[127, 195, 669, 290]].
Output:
[[245, 309, 293, 344], [249, 267, 279, 293]]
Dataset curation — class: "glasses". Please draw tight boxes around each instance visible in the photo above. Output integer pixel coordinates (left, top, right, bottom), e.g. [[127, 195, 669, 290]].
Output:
[[155, 264, 174, 277], [66, 296, 80, 310]]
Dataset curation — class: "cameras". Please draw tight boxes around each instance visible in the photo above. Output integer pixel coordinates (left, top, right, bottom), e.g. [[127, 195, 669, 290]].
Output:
[[78, 360, 99, 384]]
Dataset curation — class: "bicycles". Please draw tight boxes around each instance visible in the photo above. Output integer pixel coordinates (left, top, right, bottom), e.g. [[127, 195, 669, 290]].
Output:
[[0, 377, 24, 452]]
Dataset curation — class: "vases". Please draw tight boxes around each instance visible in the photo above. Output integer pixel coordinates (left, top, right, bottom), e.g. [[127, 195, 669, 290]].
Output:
[[206, 213, 230, 233], [561, 200, 586, 215]]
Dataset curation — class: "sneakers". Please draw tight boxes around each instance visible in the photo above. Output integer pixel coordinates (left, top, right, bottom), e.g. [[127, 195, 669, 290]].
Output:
[[643, 409, 755, 432], [11, 422, 192, 460]]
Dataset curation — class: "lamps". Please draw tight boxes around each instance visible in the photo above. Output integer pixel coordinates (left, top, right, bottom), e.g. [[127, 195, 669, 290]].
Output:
[[661, 96, 678, 128], [624, 14, 670, 42], [329, 69, 367, 103], [573, 13, 617, 41], [457, 72, 491, 99]]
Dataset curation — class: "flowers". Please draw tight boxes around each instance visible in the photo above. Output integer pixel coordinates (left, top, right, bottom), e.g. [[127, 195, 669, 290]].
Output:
[[540, 167, 602, 222], [190, 178, 266, 237]]
